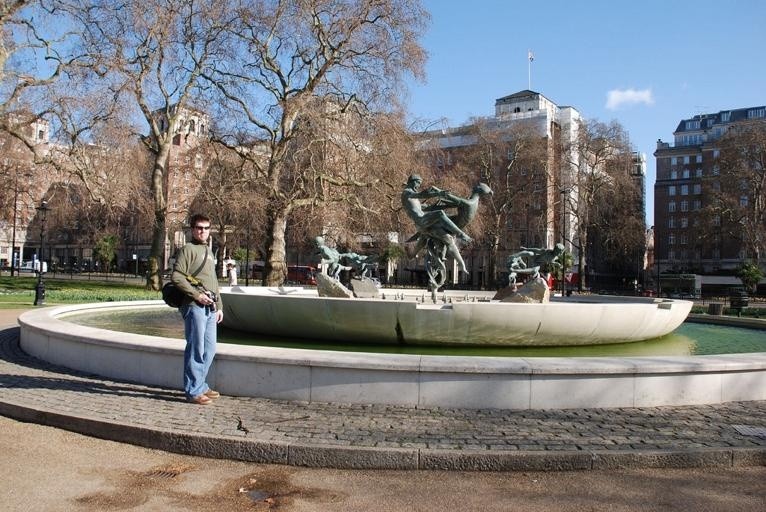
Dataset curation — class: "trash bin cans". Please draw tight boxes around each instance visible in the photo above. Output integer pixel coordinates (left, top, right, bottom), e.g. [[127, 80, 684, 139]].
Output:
[[708, 303, 723, 315]]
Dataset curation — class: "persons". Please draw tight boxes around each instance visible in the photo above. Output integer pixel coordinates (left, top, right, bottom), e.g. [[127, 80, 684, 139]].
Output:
[[401, 175, 493, 275], [226, 263, 238, 286], [311, 233, 368, 284], [506, 241, 564, 286], [171, 213, 224, 405]]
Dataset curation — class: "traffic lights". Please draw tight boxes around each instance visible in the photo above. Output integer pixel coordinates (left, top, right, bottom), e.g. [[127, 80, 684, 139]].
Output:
[[623, 242, 629, 255]]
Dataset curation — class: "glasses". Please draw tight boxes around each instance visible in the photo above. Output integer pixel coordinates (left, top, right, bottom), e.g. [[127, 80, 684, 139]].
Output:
[[196, 226, 210, 229]]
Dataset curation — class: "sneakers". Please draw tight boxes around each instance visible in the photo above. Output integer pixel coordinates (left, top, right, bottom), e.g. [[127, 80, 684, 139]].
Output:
[[191, 394, 212, 404], [205, 389, 220, 398]]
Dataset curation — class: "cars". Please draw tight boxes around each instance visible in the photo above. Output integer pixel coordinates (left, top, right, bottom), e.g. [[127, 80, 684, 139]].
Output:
[[599, 283, 657, 297], [0, 253, 173, 279]]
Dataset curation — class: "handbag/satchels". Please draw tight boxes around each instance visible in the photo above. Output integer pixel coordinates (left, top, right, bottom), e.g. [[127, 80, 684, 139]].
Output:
[[162, 282, 194, 307]]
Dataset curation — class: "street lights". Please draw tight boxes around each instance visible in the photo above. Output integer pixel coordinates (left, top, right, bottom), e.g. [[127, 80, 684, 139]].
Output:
[[560, 178, 571, 297], [11, 162, 33, 277], [128, 203, 148, 278], [233, 180, 251, 287], [290, 236, 306, 287], [647, 226, 660, 298], [33, 198, 53, 306]]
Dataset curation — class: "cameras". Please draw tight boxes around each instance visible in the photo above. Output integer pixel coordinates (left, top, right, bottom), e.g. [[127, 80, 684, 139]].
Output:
[[206, 293, 218, 313]]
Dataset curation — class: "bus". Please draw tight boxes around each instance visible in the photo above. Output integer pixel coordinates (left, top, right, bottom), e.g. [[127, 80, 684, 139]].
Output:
[[252, 264, 318, 285], [511, 273, 590, 295]]
